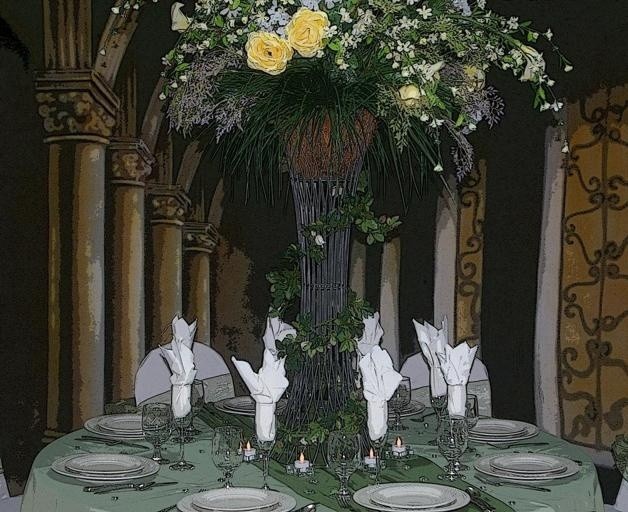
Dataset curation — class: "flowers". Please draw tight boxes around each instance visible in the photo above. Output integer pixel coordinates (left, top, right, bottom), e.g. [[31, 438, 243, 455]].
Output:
[[112, 0, 574, 442]]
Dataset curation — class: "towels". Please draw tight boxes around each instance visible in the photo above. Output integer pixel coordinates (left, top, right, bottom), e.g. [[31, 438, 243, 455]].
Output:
[[358, 346, 404, 440], [171, 315, 198, 349], [411, 315, 449, 402], [160, 342, 198, 420], [262, 307, 298, 358], [437, 338, 479, 419], [356, 313, 386, 357], [230, 352, 290, 440]]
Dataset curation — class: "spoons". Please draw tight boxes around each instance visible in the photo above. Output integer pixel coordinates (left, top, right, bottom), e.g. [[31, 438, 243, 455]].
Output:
[[73, 437, 122, 446], [94, 481, 155, 490], [294, 503, 317, 512]]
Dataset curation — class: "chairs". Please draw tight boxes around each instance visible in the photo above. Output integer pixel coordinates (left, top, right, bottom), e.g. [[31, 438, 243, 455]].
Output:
[[399, 351, 492, 417], [134, 342, 236, 412]]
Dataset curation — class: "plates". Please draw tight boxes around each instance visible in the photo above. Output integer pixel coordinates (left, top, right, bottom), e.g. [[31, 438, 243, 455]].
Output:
[[371, 483, 459, 509], [472, 450, 582, 483], [351, 482, 472, 512], [191, 488, 280, 510], [85, 412, 150, 441], [211, 393, 287, 416], [462, 416, 541, 443], [48, 453, 163, 483], [176, 486, 298, 512]]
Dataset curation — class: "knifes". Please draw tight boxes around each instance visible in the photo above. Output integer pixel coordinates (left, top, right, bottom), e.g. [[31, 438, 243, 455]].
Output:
[[81, 434, 151, 450], [81, 480, 177, 493]]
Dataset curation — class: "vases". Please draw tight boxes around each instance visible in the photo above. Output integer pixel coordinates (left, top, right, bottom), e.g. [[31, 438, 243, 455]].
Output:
[[266, 108, 382, 467]]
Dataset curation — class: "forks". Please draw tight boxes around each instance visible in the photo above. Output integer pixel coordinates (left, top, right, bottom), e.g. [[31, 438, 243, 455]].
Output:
[[474, 472, 551, 493], [486, 440, 551, 451]]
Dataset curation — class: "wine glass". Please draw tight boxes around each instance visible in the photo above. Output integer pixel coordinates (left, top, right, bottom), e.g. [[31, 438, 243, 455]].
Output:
[[327, 432, 363, 497], [387, 373, 479, 431], [437, 415, 472, 482], [254, 401, 281, 492], [211, 425, 246, 487], [366, 416, 393, 489], [141, 401, 170, 467], [170, 382, 196, 473]]
